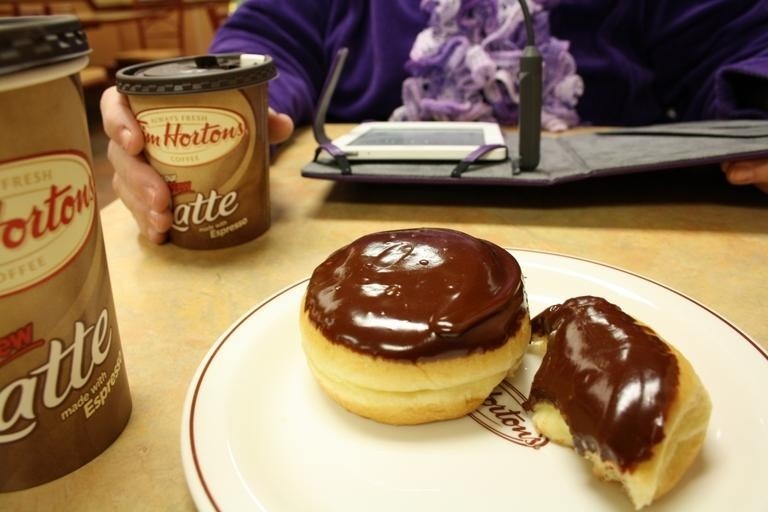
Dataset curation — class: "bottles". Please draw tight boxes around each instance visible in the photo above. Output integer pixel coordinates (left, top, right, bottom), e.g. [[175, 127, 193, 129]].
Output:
[[0, 14, 140, 495]]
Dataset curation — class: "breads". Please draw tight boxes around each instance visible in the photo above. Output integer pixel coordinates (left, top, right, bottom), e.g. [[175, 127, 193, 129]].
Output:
[[302, 226, 531, 425], [521, 294, 713, 510]]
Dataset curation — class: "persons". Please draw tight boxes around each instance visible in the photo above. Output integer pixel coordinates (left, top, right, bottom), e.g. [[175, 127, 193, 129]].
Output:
[[99, 0, 767, 245]]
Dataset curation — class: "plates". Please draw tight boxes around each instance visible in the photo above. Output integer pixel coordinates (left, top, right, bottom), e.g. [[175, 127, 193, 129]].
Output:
[[180, 245, 768, 512]]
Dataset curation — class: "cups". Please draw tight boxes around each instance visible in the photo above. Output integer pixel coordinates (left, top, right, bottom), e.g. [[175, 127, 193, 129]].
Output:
[[115, 52, 280, 252]]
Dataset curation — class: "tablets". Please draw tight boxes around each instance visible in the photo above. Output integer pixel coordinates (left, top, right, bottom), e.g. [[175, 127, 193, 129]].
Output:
[[330, 121, 508, 162]]
